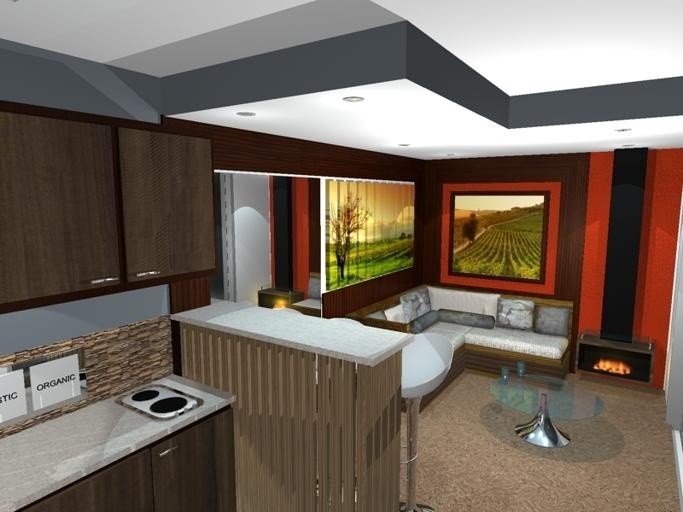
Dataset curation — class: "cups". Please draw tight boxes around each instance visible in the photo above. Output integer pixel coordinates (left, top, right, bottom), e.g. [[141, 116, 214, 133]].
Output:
[[501, 367, 509, 385], [516, 360, 526, 379]]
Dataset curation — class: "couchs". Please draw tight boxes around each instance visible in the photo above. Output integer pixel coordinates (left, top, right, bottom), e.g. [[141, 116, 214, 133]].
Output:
[[345, 284, 574, 415]]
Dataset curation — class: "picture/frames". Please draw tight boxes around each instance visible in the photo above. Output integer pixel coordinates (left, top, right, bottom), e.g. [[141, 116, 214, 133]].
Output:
[[447, 190, 550, 285]]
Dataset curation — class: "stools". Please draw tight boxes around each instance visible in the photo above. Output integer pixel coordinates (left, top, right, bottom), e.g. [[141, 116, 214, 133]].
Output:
[[400, 332, 455, 512]]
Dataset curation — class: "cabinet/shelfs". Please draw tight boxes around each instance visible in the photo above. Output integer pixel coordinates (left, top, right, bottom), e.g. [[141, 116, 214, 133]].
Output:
[[20, 404, 236, 512], [0, 110, 217, 303]]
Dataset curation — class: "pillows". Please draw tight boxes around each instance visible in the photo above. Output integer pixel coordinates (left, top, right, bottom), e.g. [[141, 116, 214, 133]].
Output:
[[365, 286, 569, 338]]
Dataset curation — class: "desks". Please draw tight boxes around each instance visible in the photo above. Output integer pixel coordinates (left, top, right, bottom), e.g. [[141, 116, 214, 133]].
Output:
[[490, 372, 604, 449]]
